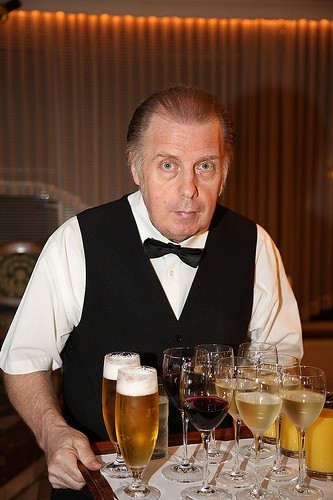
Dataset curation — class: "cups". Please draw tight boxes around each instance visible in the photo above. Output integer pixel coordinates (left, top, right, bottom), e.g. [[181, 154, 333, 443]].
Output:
[[261, 417, 278, 446], [280, 413, 306, 459], [147, 379, 169, 460], [305, 391, 333, 480]]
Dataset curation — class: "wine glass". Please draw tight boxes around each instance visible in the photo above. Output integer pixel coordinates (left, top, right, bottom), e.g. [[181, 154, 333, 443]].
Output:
[[161, 347, 210, 482], [191, 345, 234, 465], [114, 365, 160, 499], [258, 354, 300, 482], [178, 363, 232, 500], [279, 366, 326, 499], [213, 356, 257, 488], [237, 344, 279, 459], [102, 351, 140, 479], [235, 367, 283, 500]]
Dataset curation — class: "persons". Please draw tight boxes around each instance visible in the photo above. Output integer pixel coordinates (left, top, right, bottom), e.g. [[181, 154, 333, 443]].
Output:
[[0, 86, 304, 500]]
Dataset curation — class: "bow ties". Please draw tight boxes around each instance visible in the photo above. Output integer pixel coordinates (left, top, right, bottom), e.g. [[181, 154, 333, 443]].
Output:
[[143, 238, 203, 268]]
